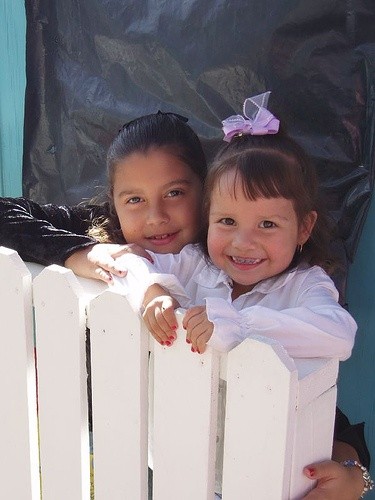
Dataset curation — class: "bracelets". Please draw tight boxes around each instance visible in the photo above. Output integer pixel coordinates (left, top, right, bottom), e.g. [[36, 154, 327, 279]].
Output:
[[338, 459, 374, 500]]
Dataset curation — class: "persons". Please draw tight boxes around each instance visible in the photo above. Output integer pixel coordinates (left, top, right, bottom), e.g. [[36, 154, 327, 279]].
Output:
[[104, 92, 357, 364], [0, 110, 375, 500]]
